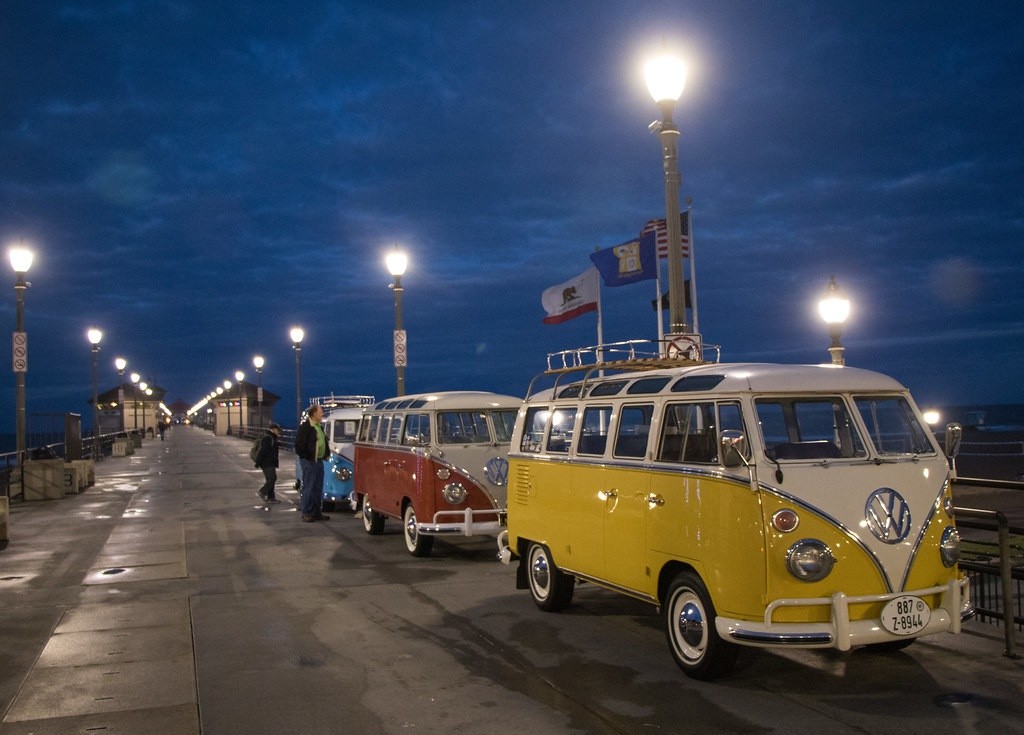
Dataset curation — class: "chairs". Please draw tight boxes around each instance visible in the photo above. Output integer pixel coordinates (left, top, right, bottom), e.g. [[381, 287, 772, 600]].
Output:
[[599, 434, 647, 457], [439, 435, 473, 444], [662, 434, 712, 462], [772, 439, 841, 459], [580, 431, 608, 455]]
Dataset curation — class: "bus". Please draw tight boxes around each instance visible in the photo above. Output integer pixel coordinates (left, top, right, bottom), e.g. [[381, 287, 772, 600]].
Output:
[[294, 392, 374, 511], [499, 334, 976, 681]]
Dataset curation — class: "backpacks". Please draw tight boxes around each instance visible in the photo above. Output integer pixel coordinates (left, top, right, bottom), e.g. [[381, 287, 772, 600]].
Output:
[[250, 435, 273, 461]]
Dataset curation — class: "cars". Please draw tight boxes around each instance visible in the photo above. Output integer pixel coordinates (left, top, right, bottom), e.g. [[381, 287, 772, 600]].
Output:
[[347, 392, 524, 555]]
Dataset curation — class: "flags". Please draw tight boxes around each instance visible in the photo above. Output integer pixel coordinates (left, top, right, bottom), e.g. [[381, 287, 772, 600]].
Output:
[[640, 210, 691, 260], [651, 278, 692, 312], [589, 233, 657, 287], [542, 264, 598, 325]]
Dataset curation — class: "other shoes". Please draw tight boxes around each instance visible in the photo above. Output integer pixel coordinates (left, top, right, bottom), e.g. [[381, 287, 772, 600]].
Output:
[[268, 498, 280, 504], [302, 513, 314, 522], [314, 512, 330, 521], [257, 490, 266, 502]]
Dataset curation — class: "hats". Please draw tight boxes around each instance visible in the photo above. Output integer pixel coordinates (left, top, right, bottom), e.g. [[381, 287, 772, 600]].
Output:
[[270, 423, 283, 432]]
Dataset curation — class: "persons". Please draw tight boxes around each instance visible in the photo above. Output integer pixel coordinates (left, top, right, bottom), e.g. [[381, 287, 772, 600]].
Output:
[[257, 424, 282, 503], [295, 405, 331, 522], [158, 419, 165, 441]]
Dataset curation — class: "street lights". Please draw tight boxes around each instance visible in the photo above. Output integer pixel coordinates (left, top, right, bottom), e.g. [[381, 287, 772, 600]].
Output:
[[224, 381, 233, 436], [115, 358, 125, 430], [630, 33, 696, 334], [131, 373, 141, 429], [236, 371, 244, 438], [384, 249, 412, 396], [141, 383, 153, 439], [824, 275, 855, 451], [253, 356, 264, 427], [290, 328, 303, 427], [87, 329, 101, 461], [8, 247, 31, 504]]
